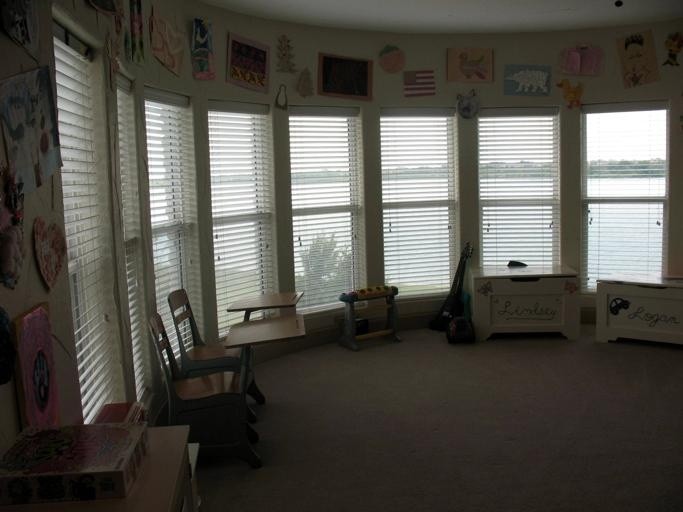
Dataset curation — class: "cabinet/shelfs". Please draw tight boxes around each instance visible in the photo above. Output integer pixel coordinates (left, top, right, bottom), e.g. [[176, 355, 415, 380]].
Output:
[[464, 265, 683, 347], [1, 420, 204, 512]]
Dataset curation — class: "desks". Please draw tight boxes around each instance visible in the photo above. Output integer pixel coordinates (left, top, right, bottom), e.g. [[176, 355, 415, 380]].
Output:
[[219, 289, 307, 388]]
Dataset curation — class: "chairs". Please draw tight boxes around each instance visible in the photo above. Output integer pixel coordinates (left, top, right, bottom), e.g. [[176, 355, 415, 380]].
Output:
[[147, 288, 267, 470]]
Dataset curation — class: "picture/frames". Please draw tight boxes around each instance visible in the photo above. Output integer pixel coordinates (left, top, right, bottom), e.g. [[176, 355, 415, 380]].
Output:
[[223, 34, 378, 102]]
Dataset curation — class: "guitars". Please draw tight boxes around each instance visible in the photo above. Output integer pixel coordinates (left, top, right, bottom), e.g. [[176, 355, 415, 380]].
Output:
[[432, 241, 475, 332]]
